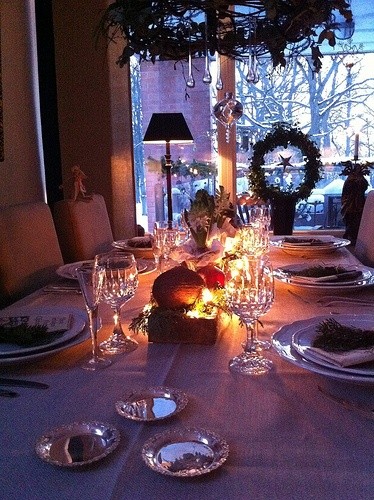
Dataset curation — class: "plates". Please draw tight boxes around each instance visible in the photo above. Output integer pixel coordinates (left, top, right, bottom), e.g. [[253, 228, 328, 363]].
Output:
[[34, 420, 121, 469], [268, 235, 374, 386], [115, 386, 189, 423], [0, 307, 103, 366], [55, 236, 157, 282], [141, 425, 231, 478]]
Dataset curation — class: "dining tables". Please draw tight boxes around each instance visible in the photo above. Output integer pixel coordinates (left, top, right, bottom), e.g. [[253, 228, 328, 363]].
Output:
[[0, 230, 373, 500]]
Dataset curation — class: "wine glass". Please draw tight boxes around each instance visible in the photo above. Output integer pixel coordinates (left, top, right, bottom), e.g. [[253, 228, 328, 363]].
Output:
[[75, 266, 113, 371], [150, 209, 192, 276], [222, 207, 275, 376], [94, 251, 140, 356]]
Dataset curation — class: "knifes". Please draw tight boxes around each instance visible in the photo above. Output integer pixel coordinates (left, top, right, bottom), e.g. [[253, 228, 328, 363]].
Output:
[[0, 389, 17, 398], [0, 378, 49, 389]]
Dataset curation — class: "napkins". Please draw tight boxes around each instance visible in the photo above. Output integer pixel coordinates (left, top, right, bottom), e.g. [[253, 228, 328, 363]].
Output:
[[79, 261, 129, 272], [305, 321, 374, 369], [282, 237, 340, 248], [281, 263, 361, 282], [0, 312, 72, 346], [127, 235, 155, 248]]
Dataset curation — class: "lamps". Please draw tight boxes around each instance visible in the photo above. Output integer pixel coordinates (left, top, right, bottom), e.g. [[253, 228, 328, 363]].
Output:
[[141, 113, 194, 228]]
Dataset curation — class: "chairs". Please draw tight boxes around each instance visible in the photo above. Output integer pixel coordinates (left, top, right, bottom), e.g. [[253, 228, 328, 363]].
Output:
[[352, 190, 374, 270], [53, 195, 114, 264], [0, 198, 64, 311]]
